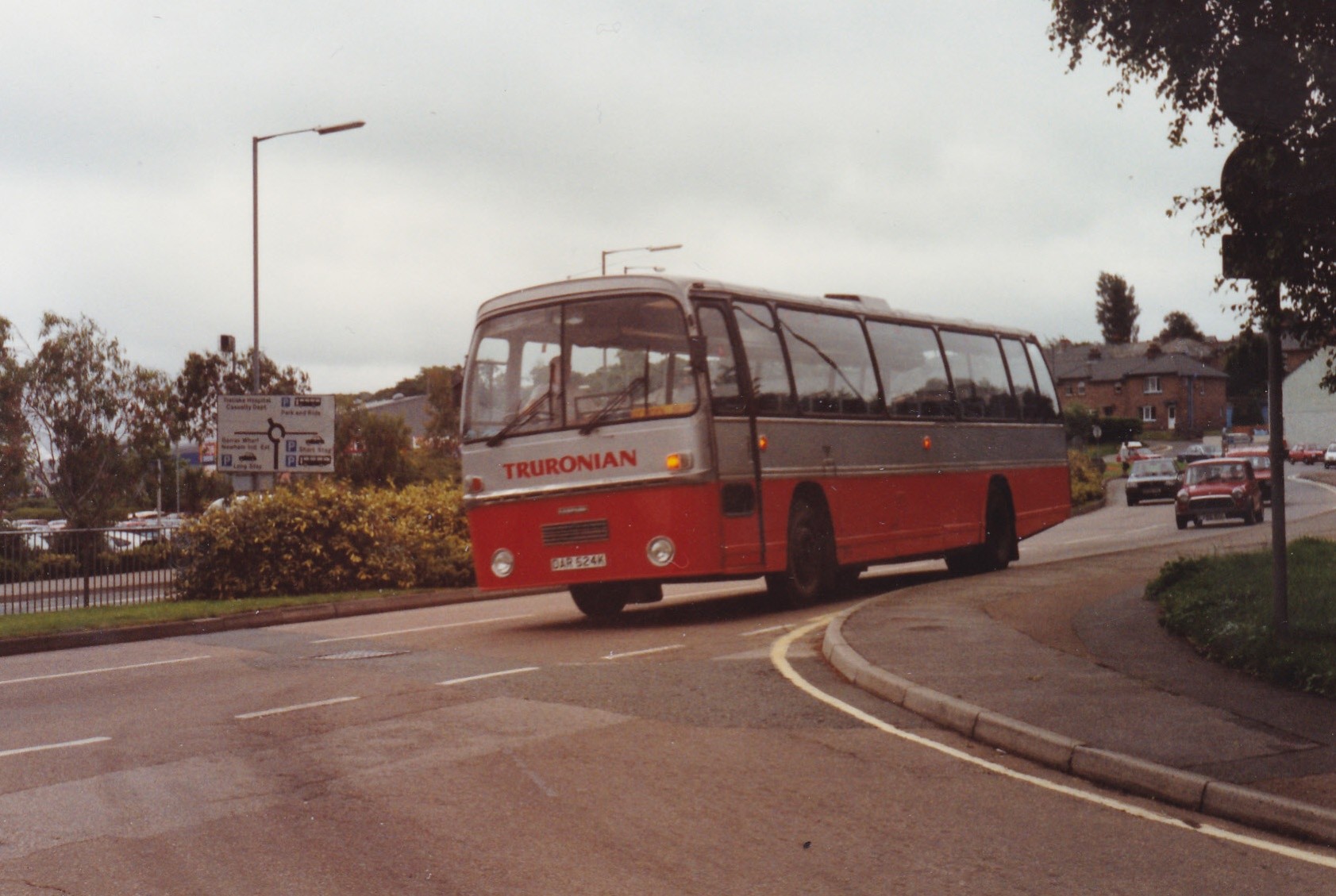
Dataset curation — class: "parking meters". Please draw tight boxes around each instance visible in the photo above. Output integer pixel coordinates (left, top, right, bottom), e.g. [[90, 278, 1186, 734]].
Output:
[[254, 121, 366, 394]]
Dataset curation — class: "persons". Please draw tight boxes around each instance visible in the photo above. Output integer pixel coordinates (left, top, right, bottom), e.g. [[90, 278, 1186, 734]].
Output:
[[520, 356, 577, 418]]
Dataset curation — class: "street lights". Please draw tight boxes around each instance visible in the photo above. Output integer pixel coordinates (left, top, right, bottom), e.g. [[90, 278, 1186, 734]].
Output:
[[624, 266, 666, 276], [602, 243, 683, 371], [221, 334, 236, 383]]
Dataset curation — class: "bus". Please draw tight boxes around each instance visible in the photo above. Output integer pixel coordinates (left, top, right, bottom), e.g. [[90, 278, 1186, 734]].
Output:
[[459, 271, 1071, 617]]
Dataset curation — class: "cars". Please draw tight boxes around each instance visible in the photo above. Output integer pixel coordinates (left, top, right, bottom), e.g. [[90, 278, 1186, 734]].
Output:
[[1178, 444, 1222, 464], [1225, 445, 1273, 506], [1125, 458, 1186, 506], [1287, 442, 1324, 465], [1324, 442, 1336, 469], [13, 510, 184, 553], [1174, 458, 1263, 529]]
